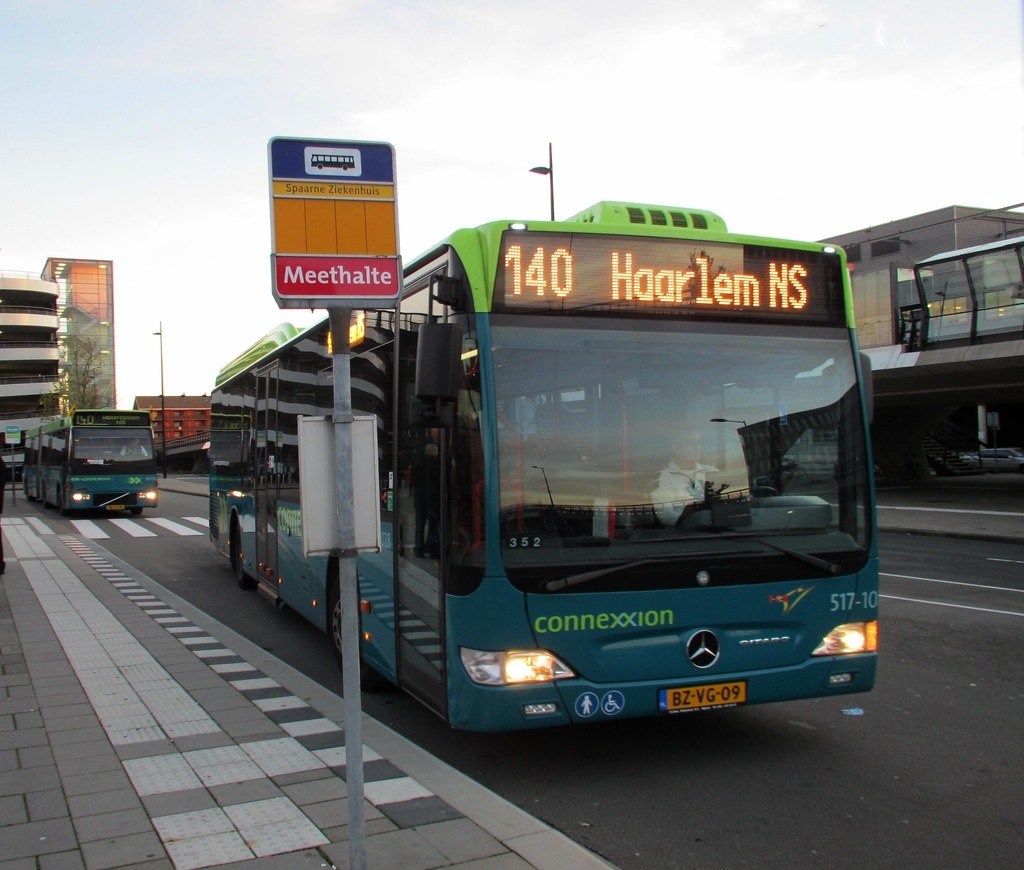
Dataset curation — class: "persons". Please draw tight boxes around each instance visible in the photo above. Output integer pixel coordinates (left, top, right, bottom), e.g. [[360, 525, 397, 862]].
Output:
[[0, 456, 7, 575], [121, 435, 148, 456], [410, 444, 445, 560], [652, 423, 725, 527]]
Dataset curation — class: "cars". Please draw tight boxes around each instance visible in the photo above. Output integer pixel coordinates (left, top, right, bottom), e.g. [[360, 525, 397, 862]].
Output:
[[962, 447, 1024, 476]]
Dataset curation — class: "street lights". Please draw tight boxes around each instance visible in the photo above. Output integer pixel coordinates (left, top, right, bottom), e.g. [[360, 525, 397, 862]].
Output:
[[528, 142, 558, 220], [153, 320, 168, 479]]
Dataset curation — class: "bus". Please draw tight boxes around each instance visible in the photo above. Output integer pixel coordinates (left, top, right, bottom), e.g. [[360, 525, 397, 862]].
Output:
[[22, 408, 159, 517], [203, 198, 883, 736]]
